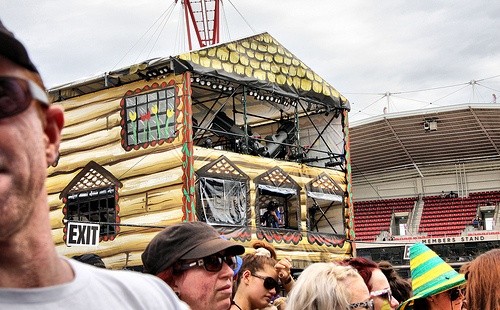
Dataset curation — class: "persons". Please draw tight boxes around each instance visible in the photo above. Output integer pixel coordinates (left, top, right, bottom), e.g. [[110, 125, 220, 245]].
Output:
[[440, 191, 446, 198], [260, 200, 282, 228], [141, 221, 245, 310], [0, 16, 191, 310], [399, 241, 467, 310], [449, 191, 455, 196], [472, 216, 480, 223], [229, 241, 412, 310], [72, 253, 106, 268], [459, 248, 500, 310]]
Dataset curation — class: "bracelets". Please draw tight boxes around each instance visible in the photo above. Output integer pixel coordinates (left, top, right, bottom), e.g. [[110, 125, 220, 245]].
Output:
[[281, 275, 292, 285]]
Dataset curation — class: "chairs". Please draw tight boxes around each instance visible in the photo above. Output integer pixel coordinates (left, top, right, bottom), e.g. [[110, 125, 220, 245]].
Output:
[[353, 190, 500, 242]]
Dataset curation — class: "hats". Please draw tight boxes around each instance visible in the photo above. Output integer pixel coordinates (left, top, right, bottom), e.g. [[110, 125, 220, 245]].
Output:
[[0, 20, 38, 71], [71, 253, 106, 269], [407, 242, 467, 301], [141, 220, 246, 275]]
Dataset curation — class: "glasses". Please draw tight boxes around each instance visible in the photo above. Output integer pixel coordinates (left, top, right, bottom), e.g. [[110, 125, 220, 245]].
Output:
[[434, 287, 467, 301], [171, 254, 235, 275], [336, 299, 373, 310], [369, 288, 393, 302], [0, 76, 49, 119], [240, 274, 280, 297], [465, 271, 470, 281]]
